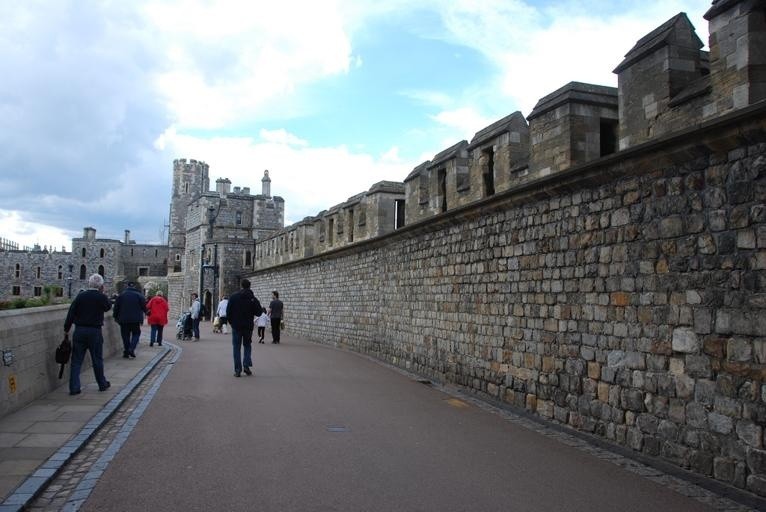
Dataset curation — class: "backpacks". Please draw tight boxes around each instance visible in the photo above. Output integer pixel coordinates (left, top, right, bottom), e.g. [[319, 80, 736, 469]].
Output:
[[199, 303, 209, 316]]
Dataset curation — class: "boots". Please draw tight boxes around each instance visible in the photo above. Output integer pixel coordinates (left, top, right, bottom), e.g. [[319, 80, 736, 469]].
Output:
[[222, 323, 228, 334]]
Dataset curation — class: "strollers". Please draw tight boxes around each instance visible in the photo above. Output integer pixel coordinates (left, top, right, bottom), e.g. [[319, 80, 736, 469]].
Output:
[[175, 311, 192, 341], [211, 311, 227, 334]]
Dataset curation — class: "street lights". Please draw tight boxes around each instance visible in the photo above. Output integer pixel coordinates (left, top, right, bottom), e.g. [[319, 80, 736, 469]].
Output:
[[65, 274, 73, 297]]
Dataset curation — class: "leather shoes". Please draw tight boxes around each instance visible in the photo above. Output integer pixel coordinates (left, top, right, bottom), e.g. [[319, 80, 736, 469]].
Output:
[[70, 389, 81, 395], [99, 381, 110, 391], [244, 368, 252, 375], [128, 351, 136, 357], [233, 373, 240, 377], [122, 354, 129, 358]]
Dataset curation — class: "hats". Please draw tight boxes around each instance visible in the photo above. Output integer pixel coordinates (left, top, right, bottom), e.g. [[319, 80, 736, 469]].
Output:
[[127, 281, 135, 287]]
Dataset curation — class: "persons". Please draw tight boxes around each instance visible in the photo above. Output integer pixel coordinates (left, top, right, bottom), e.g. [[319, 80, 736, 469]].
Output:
[[212, 295, 228, 333], [145, 290, 169, 347], [226, 280, 263, 377], [64, 274, 112, 395], [254, 307, 270, 344], [188, 293, 202, 343], [267, 291, 284, 344], [113, 281, 151, 358]]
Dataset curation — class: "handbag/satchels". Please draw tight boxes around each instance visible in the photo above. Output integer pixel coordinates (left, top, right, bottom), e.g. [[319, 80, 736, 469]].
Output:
[[55, 335, 72, 379]]
